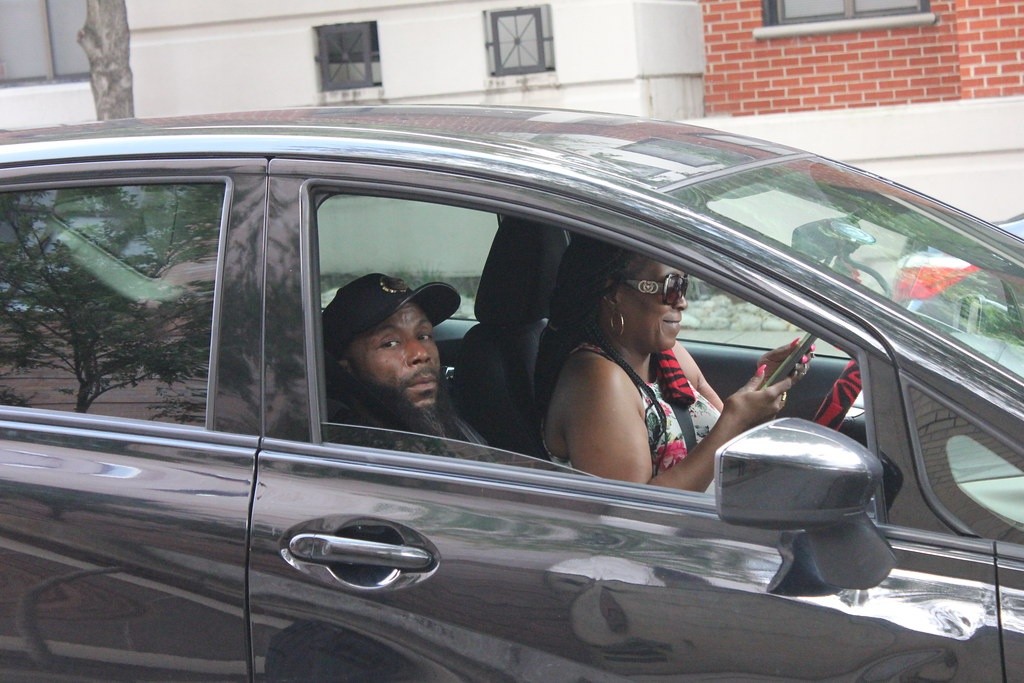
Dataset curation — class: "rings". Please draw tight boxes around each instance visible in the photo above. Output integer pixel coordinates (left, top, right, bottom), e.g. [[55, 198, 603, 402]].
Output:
[[782, 392, 787, 402], [801, 363, 807, 375]]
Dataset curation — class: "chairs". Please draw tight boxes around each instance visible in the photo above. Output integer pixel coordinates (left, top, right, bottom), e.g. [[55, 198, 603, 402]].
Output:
[[460, 216, 571, 463]]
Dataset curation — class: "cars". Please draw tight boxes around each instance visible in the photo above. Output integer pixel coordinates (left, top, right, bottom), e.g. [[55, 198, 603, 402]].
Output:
[[893, 211, 1024, 312], [1, 103, 1024, 683]]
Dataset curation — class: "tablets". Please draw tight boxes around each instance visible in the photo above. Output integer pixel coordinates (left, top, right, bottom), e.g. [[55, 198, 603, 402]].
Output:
[[760, 331, 815, 390]]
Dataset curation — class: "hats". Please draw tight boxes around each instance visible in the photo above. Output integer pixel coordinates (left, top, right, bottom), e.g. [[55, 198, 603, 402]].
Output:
[[322, 273, 460, 344]]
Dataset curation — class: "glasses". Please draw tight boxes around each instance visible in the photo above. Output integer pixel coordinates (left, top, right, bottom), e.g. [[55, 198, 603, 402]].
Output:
[[626, 272, 690, 304]]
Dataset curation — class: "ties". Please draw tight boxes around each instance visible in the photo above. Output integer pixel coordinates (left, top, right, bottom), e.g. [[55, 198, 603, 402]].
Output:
[[449, 413, 497, 463]]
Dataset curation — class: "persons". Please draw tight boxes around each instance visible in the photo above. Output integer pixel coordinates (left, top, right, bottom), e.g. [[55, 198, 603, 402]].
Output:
[[537, 237, 814, 494], [320, 273, 535, 469]]
